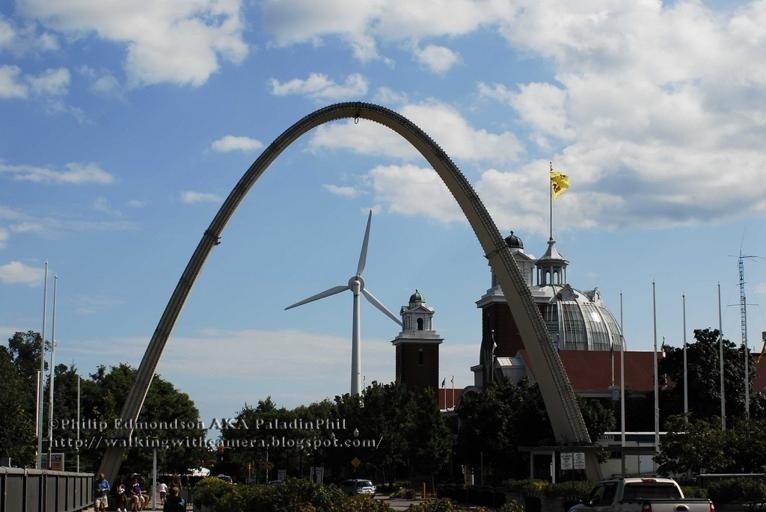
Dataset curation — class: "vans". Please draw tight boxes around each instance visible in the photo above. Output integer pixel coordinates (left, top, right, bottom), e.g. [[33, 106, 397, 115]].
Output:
[[339, 479, 376, 498]]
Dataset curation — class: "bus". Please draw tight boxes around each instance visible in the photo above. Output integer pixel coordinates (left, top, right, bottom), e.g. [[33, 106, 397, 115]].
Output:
[[159, 474, 233, 502]]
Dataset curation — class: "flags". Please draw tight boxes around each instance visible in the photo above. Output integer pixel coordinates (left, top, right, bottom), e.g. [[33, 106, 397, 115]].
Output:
[[442, 378, 446, 387], [551, 169, 570, 199]]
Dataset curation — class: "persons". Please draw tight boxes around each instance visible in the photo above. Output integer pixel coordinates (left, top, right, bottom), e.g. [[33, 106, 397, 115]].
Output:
[[129, 477, 145, 512], [91, 473, 110, 511], [109, 476, 127, 512], [172, 475, 182, 496], [157, 478, 168, 506], [163, 486, 186, 511]]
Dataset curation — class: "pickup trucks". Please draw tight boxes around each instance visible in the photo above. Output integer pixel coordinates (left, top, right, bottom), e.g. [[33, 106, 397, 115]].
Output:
[[567, 477, 715, 512]]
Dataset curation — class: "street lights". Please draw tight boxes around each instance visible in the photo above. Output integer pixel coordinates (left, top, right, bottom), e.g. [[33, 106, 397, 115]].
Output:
[[150, 436, 160, 510], [265, 443, 271, 483]]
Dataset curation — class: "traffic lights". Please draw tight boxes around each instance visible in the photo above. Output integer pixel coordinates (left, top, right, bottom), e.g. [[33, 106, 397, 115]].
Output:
[[219, 444, 224, 455]]
